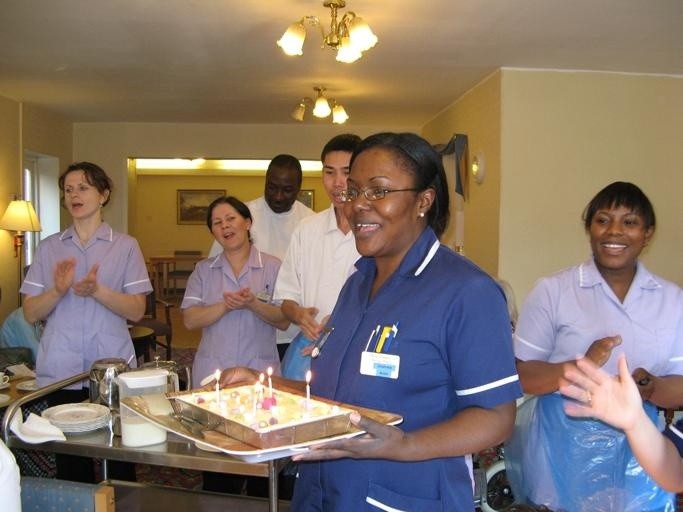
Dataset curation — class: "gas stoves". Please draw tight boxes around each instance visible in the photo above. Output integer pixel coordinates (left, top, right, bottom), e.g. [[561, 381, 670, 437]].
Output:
[[175, 383, 353, 433]]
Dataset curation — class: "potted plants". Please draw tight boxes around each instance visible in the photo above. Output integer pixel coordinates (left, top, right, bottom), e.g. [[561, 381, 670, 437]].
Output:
[[297, 189, 315, 211], [176, 189, 226, 225]]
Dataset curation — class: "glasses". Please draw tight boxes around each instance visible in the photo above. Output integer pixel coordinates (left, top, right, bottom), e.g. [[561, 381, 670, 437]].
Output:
[[275, 0, 378, 65], [288, 87, 348, 126], [0, 199, 42, 256]]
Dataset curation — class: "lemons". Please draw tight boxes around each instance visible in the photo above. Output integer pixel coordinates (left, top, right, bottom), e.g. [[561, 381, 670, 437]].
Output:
[[89, 356, 131, 408], [137, 355, 191, 394]]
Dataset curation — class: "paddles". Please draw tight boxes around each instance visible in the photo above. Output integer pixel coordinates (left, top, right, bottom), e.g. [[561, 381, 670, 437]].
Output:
[[136, 345, 206, 490]]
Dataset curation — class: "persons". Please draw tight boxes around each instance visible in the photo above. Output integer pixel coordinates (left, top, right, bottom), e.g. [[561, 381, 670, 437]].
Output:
[[0, 306, 45, 367], [17, 160, 155, 485], [557, 351, 683, 495], [510, 180, 682, 511], [178, 196, 290, 498], [208, 153, 317, 361], [272, 132, 364, 384], [209, 129, 524, 512]]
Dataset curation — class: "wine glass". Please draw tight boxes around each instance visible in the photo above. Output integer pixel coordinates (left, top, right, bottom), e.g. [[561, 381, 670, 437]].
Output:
[[586, 393, 592, 405]]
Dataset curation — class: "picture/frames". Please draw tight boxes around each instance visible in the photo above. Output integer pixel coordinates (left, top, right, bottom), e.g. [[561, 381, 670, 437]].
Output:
[[150, 253, 207, 299]]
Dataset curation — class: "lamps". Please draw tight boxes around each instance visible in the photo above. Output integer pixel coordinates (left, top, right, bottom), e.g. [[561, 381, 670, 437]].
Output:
[[139, 278, 174, 360], [20, 477, 116, 512]]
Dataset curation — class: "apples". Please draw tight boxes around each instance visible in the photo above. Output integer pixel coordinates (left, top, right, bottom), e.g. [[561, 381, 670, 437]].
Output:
[[304, 369, 312, 402], [267, 365, 274, 396], [251, 381, 262, 417], [215, 369, 223, 398]]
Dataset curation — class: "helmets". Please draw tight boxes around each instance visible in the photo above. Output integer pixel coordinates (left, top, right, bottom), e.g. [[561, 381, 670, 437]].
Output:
[[42, 403, 110, 432], [0, 393, 9, 402], [16, 380, 37, 391], [0, 382, 10, 388]]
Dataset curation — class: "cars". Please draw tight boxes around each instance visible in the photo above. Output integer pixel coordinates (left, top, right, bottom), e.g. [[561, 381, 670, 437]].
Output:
[[0, 372, 9, 385]]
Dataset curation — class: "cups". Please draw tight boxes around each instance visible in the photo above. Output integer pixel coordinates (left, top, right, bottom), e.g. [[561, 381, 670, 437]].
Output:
[[340, 187, 417, 202]]
